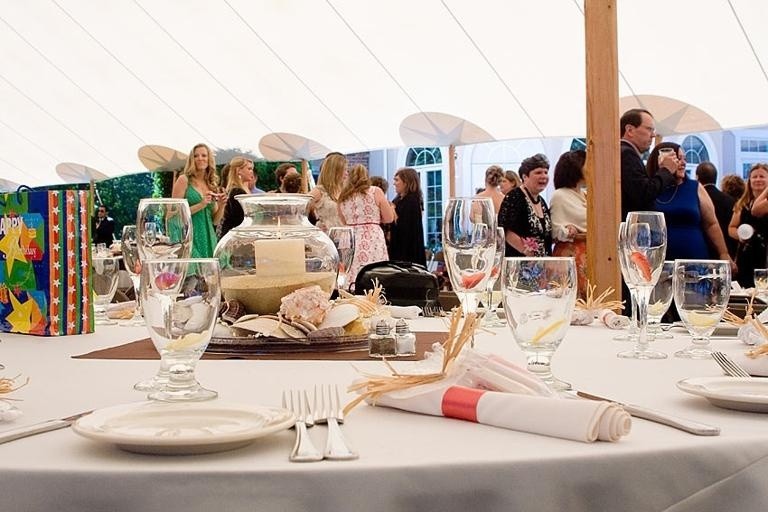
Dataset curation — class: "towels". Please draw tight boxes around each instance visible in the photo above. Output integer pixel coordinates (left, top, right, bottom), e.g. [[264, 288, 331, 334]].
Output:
[[598, 308, 631, 329], [354, 375, 636, 446]]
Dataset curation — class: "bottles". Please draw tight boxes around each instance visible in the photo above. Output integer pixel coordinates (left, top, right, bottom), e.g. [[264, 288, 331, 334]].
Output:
[[368, 319, 395, 358], [394, 319, 416, 357]]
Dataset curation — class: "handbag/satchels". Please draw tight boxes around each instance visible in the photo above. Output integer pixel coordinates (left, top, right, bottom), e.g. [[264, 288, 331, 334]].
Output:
[[349, 260, 438, 305], [0, 186, 94, 338]]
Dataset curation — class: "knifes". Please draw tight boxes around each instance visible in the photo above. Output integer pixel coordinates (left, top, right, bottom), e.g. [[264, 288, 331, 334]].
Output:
[[0, 407, 95, 447], [576, 391, 721, 436]]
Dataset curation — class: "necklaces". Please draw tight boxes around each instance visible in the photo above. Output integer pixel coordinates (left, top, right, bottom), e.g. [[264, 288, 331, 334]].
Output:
[[654, 182, 679, 205], [523, 184, 541, 205]]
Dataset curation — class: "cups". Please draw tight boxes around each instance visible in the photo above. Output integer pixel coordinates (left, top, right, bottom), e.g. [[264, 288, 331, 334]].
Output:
[[91, 237, 121, 258], [658, 148, 674, 162]]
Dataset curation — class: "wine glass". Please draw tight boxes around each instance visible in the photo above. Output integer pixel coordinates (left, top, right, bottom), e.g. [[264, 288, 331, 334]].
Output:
[[616, 211, 668, 359], [441, 196, 497, 350], [629, 261, 675, 339], [471, 226, 508, 328], [118, 225, 147, 327], [754, 269, 768, 300], [500, 257, 578, 391], [327, 226, 356, 301], [91, 257, 120, 325], [133, 198, 194, 390], [139, 258, 222, 401], [612, 221, 651, 341], [672, 259, 732, 360]]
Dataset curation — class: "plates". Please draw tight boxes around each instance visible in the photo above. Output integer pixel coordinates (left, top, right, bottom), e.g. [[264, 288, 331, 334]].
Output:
[[71, 399, 297, 456], [672, 320, 739, 336], [675, 375, 768, 414], [730, 288, 752, 297]]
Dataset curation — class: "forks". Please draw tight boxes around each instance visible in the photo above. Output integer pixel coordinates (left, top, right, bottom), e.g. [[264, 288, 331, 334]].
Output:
[[711, 350, 751, 377], [721, 309, 744, 328], [281, 389, 324, 461], [421, 306, 451, 318], [312, 383, 359, 460]]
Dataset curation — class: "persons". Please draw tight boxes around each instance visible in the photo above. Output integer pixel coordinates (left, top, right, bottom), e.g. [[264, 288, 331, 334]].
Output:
[[266, 163, 299, 193], [500, 169, 520, 196], [475, 186, 485, 195], [497, 153, 578, 294], [370, 175, 388, 196], [549, 149, 588, 306], [167, 143, 234, 298], [750, 185, 768, 216], [92, 205, 115, 249], [720, 175, 745, 200], [728, 163, 768, 288], [620, 108, 679, 323], [246, 167, 263, 193], [391, 167, 428, 272], [219, 157, 254, 240], [278, 172, 306, 194], [214, 166, 231, 241], [469, 165, 505, 225], [308, 156, 349, 237], [336, 164, 397, 293], [642, 142, 739, 321], [694, 160, 736, 288], [320, 152, 346, 172]]
